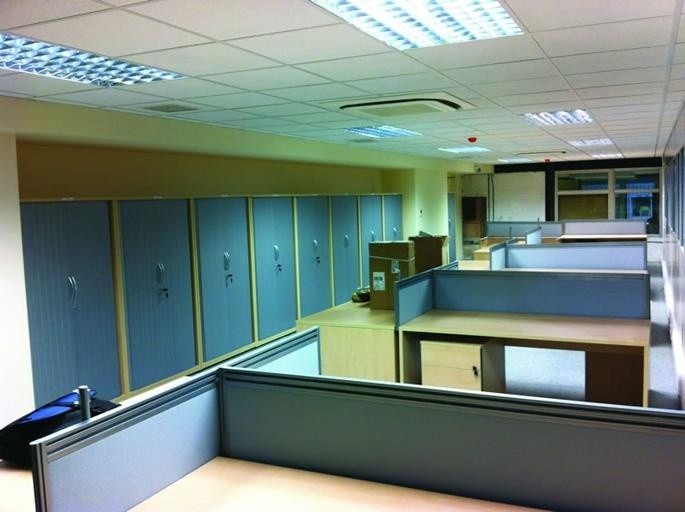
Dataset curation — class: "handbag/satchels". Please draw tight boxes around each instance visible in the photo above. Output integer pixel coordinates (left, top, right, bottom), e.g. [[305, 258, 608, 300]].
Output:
[[0, 389, 120, 467]]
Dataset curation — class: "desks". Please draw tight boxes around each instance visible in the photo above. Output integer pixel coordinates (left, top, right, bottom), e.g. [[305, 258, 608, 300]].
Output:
[[0, 375, 198, 512], [296, 235, 651, 408], [127, 456, 555, 512]]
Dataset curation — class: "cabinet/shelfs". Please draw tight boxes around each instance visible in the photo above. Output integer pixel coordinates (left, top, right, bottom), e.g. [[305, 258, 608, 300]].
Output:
[[115, 196, 203, 398], [359, 193, 403, 291], [329, 194, 362, 309], [193, 196, 260, 370], [249, 195, 300, 346], [294, 194, 334, 321], [21, 197, 127, 414]]
